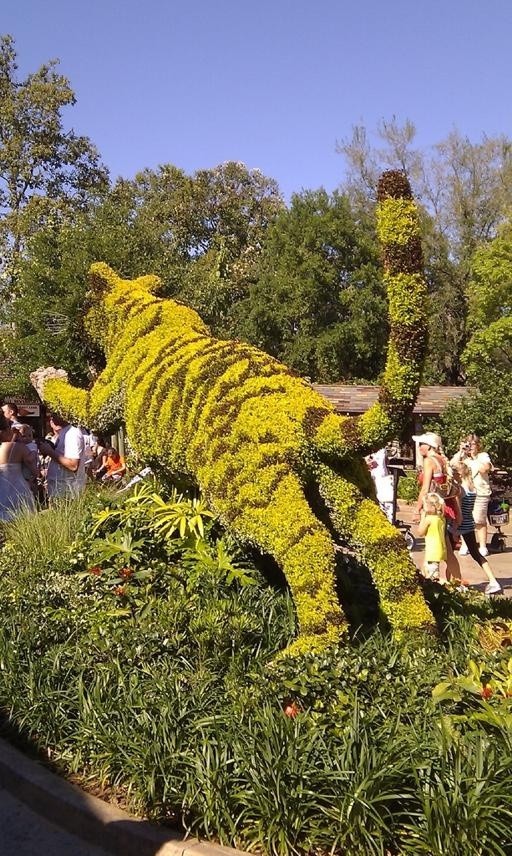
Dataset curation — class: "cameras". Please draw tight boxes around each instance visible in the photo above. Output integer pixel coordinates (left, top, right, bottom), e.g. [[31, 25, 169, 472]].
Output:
[[464, 444, 470, 449]]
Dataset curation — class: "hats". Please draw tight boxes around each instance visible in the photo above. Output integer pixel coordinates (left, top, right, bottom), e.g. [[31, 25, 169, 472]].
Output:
[[412, 432, 441, 447]]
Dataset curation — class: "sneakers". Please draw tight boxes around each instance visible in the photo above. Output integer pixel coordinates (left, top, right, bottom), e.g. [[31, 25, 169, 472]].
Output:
[[484, 583, 501, 594]]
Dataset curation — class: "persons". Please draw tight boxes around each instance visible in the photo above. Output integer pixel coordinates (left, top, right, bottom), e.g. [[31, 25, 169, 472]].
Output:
[[0, 402, 123, 523], [365, 431, 503, 594]]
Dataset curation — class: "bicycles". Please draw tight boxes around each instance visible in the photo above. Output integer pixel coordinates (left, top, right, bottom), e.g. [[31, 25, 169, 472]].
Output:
[[388, 518, 418, 551]]
